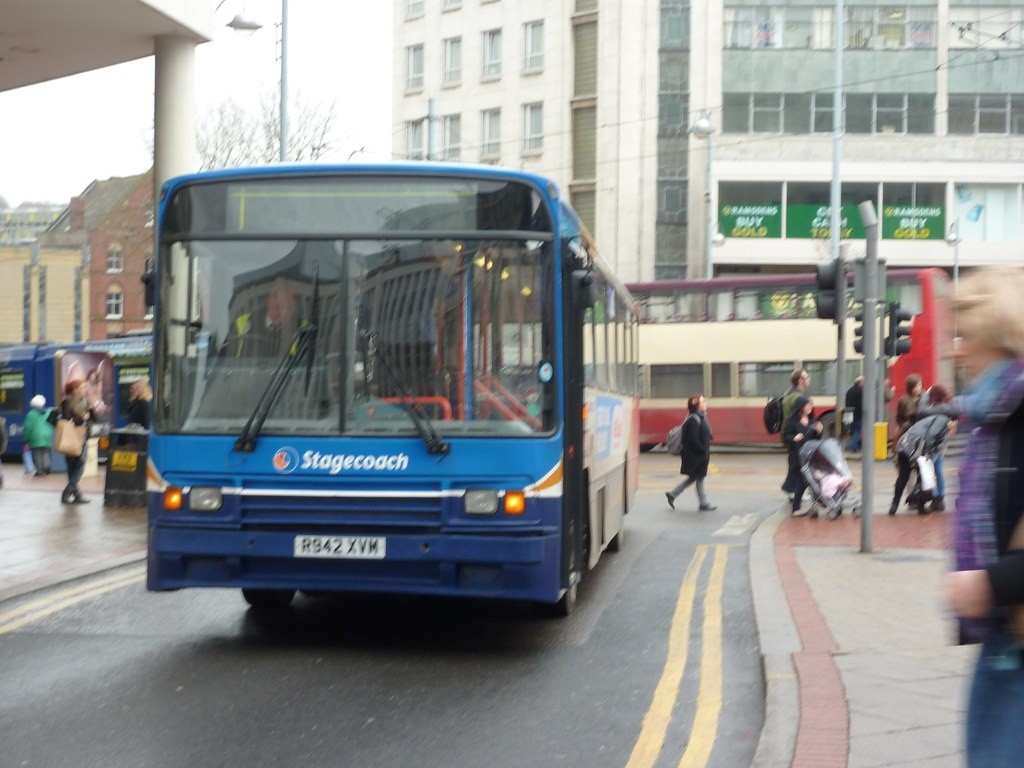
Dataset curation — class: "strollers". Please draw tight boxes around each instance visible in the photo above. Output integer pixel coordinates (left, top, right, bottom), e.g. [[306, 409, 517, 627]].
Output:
[[796, 428, 861, 522]]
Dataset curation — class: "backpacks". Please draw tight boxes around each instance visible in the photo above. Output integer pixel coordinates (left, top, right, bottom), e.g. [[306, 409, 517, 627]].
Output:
[[764, 388, 796, 434], [667, 412, 701, 455]]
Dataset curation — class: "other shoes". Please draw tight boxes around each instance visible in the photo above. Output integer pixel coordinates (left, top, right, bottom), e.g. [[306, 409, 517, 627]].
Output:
[[700, 503, 717, 511], [667, 492, 676, 510]]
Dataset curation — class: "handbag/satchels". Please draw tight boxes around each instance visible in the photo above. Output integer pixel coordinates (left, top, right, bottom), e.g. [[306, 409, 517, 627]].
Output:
[[22, 443, 34, 474], [47, 403, 63, 425], [55, 416, 86, 457]]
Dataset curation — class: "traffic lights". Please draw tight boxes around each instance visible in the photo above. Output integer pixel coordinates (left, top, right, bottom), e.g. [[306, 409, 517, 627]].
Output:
[[888, 301, 913, 357], [853, 311, 865, 354], [815, 254, 840, 324]]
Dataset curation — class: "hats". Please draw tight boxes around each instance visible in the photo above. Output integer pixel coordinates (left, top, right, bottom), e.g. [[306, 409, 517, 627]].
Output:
[[793, 396, 809, 416]]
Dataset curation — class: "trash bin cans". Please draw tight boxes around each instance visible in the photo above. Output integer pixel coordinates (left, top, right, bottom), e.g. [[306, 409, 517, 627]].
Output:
[[104, 427, 151, 510]]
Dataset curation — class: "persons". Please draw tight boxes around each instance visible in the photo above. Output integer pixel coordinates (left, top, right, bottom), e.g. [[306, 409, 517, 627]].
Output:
[[84, 369, 99, 406], [780, 368, 811, 503], [231, 277, 308, 357], [845, 375, 865, 453], [61, 381, 91, 504], [781, 395, 823, 517], [126, 379, 153, 428], [665, 393, 718, 511], [945, 306, 1024, 768], [21, 394, 52, 476], [876, 376, 896, 422], [889, 374, 951, 516]]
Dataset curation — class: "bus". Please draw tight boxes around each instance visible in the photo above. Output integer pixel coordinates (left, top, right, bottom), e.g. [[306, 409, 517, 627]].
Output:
[[0, 333, 277, 480], [468, 265, 957, 454], [140, 160, 642, 620]]
